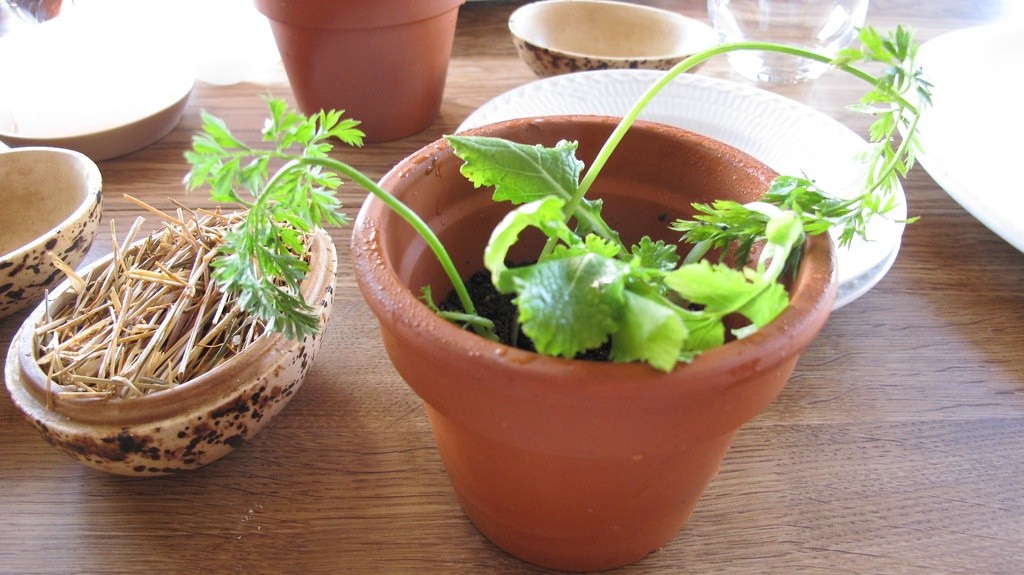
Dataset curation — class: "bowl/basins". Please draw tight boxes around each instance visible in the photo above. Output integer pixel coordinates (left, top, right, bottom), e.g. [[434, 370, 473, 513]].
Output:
[[507, 0, 720, 77], [0, 145, 103, 319]]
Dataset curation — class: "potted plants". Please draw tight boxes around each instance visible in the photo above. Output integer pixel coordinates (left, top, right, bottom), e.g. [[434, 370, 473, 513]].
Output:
[[180, 18, 932, 570]]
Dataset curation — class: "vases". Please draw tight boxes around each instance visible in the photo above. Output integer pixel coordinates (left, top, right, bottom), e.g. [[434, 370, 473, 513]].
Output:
[[252, 1, 467, 146]]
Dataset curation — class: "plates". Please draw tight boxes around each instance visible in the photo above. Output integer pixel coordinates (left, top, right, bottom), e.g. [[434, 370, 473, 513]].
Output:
[[450, 69, 908, 314], [0, 23, 198, 156], [891, 19, 1024, 258]]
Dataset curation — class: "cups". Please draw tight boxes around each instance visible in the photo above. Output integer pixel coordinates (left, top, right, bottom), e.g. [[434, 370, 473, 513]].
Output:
[[705, 0, 870, 84]]
